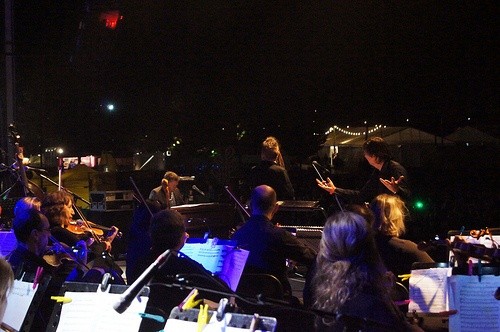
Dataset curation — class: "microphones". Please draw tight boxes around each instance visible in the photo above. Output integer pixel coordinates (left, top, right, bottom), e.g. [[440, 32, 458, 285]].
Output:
[[24, 166, 47, 172], [192, 184, 206, 196], [312, 161, 330, 172], [112, 248, 172, 312]]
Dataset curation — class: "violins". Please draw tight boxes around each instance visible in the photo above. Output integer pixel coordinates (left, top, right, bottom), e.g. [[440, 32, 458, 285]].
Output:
[[65, 218, 115, 235]]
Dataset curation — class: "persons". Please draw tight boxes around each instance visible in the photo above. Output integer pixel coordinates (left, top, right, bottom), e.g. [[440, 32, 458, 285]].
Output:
[[124, 209, 229, 293], [316, 135, 413, 205], [232, 184, 315, 297], [239, 137, 295, 200], [308, 212, 425, 332], [0, 258, 18, 332], [367, 193, 434, 291], [3, 190, 111, 277], [148, 172, 184, 208]]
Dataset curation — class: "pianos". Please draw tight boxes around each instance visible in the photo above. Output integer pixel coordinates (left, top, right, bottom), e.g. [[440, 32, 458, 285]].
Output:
[[168, 202, 238, 238]]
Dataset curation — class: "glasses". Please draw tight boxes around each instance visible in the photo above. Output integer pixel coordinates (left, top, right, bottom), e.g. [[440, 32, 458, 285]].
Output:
[[43, 228, 52, 231]]
